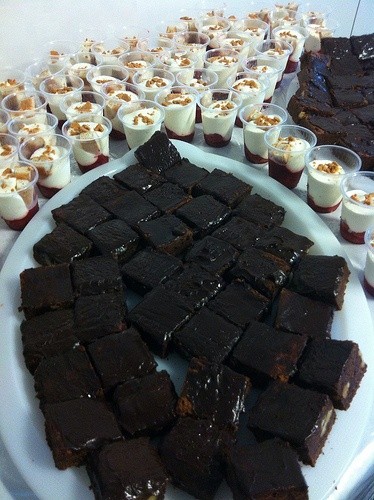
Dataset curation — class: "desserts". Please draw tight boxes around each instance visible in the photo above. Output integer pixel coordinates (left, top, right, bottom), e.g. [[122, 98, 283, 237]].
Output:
[[286, 33, 374, 173], [20, 130, 369, 500]]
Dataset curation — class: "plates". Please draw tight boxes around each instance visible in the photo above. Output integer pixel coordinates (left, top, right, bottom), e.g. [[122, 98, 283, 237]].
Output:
[[0, 138, 374, 500]]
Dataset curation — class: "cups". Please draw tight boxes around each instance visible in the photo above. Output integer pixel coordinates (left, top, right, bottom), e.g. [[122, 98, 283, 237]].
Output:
[[59, 90, 106, 115], [86, 64, 129, 93], [227, 72, 270, 128], [264, 125, 317, 190], [20, 133, 73, 199], [154, 86, 197, 143], [157, 2, 337, 103], [117, 98, 166, 150], [100, 81, 146, 141], [0, 134, 40, 231], [239, 102, 288, 164], [176, 68, 219, 124], [61, 114, 112, 174], [0, 39, 83, 137], [117, 50, 158, 81], [90, 38, 130, 66], [65, 52, 103, 90], [306, 145, 374, 298], [136, 35, 174, 58], [197, 89, 241, 147], [132, 68, 176, 101]]
